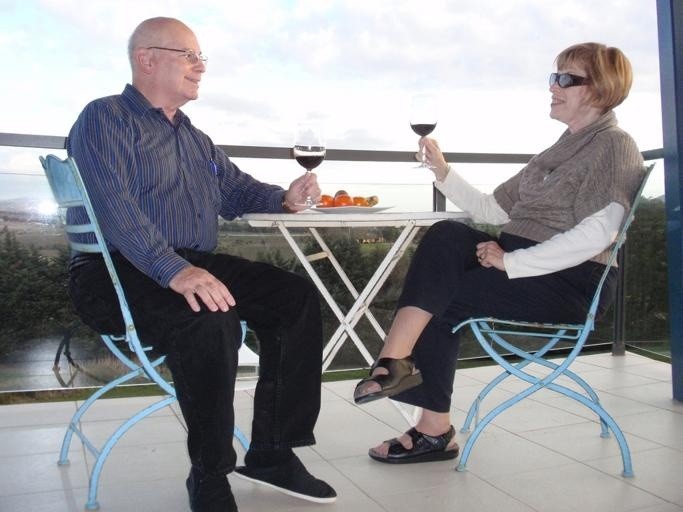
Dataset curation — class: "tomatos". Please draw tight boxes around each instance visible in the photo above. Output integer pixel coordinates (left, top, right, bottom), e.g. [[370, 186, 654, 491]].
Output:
[[318, 189, 378, 207]]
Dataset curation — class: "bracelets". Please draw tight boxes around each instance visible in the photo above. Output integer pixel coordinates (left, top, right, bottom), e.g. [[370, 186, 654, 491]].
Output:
[[280, 191, 297, 213], [435, 163, 450, 182]]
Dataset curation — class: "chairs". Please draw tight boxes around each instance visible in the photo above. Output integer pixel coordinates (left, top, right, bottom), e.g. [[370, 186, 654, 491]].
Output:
[[37, 155, 249, 512], [449, 162, 656, 479]]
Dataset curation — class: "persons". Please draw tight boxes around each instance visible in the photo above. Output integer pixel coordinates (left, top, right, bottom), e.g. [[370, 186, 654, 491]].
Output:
[[65, 16, 337, 512], [354, 42, 644, 464]]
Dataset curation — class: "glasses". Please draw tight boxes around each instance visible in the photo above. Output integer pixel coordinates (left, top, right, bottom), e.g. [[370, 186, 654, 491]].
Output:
[[146, 46, 208, 65], [549, 72, 592, 88]]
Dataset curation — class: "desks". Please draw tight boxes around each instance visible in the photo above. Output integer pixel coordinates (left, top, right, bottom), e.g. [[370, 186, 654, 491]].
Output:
[[242, 207, 472, 429]]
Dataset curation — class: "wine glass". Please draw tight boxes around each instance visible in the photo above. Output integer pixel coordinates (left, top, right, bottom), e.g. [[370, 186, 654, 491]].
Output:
[[294, 141, 326, 206], [409, 116, 438, 168]]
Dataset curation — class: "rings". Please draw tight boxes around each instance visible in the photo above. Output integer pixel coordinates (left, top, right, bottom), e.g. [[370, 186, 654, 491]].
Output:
[[479, 256, 484, 261], [195, 284, 201, 294]]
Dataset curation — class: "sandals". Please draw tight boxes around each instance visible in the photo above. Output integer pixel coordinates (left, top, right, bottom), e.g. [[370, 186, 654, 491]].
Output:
[[354, 355, 424, 404], [368, 425, 459, 463]]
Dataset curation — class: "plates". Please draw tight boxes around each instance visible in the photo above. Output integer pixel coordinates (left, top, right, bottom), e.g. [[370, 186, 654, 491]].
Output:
[[314, 207, 391, 214]]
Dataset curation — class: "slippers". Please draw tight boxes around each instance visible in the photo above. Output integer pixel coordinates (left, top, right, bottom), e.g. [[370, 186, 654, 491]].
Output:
[[233, 455, 336, 503], [186, 466, 237, 512]]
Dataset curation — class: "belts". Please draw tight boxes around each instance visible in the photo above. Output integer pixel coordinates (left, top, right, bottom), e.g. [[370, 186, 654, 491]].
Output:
[[68, 253, 100, 273]]
